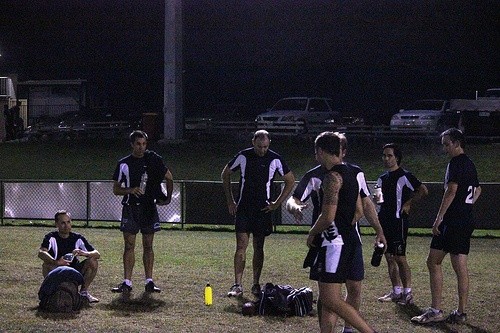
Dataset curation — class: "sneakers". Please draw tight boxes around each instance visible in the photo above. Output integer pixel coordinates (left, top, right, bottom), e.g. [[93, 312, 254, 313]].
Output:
[[445, 312, 468, 322], [395, 291, 415, 306], [80, 292, 99, 303], [251, 284, 263, 296], [228, 284, 242, 297], [410, 309, 444, 324], [378, 292, 402, 302], [112, 282, 133, 293], [145, 281, 161, 292]]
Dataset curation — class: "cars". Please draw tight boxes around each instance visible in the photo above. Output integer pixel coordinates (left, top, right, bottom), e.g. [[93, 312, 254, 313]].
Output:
[[184, 112, 214, 138], [389, 99, 450, 136], [57, 106, 142, 150]]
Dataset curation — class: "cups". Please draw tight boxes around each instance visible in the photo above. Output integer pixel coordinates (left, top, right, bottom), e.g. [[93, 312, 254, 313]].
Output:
[[371, 241, 385, 267]]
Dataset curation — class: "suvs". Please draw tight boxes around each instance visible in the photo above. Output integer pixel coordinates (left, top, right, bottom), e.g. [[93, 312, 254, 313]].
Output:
[[254, 96, 335, 137]]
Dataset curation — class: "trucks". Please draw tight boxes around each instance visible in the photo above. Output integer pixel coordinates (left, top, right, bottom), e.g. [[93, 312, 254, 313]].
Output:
[[449, 87, 500, 136]]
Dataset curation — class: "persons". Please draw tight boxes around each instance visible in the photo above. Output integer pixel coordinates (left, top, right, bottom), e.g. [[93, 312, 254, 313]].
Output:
[[286, 131, 388, 333], [221, 130, 295, 295], [39, 211, 102, 302], [372, 143, 428, 303], [410, 128, 480, 324], [109, 130, 174, 293]]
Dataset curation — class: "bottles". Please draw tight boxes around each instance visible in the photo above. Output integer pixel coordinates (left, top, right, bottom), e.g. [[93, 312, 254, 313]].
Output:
[[374, 184, 384, 203], [140, 171, 147, 194], [205, 284, 212, 305]]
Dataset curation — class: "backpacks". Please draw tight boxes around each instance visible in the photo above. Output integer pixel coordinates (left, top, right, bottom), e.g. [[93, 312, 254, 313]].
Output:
[[38, 280, 78, 312]]
